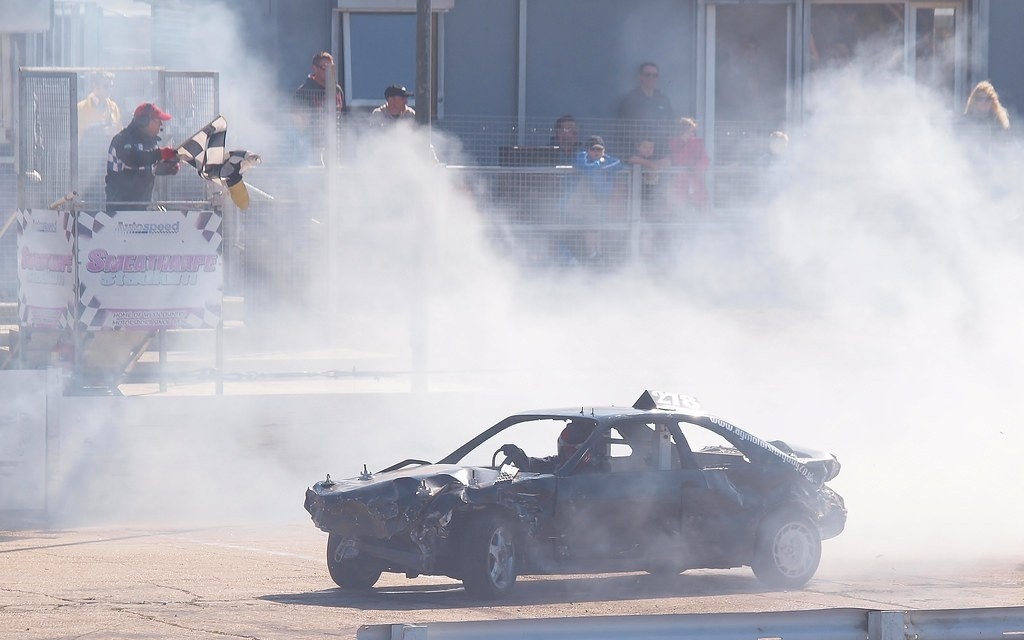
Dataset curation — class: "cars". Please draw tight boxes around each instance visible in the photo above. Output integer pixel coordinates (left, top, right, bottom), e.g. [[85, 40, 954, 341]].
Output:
[[303, 394, 848, 602]]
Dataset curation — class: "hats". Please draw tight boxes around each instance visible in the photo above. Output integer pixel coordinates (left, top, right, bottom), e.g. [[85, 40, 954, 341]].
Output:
[[384, 84, 414, 101], [135, 104, 172, 121], [586, 136, 604, 150]]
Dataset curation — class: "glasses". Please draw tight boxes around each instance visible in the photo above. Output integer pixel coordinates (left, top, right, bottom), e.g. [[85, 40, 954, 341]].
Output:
[[589, 147, 603, 152], [641, 72, 659, 78], [315, 64, 328, 70], [976, 97, 994, 104]]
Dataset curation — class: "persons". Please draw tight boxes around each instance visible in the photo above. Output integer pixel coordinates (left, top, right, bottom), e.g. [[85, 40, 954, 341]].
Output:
[[77, 72, 120, 133], [958, 80, 1011, 137], [153, 76, 199, 136], [754, 131, 790, 165], [500, 423, 612, 477], [291, 51, 344, 163], [546, 63, 710, 267], [370, 84, 415, 132], [105, 102, 176, 213]]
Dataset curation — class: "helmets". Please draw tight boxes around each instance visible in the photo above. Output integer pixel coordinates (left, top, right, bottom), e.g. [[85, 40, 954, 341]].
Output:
[[558, 423, 605, 467]]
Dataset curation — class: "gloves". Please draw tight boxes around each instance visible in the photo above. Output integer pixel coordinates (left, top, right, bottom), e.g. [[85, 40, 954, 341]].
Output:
[[502, 444, 527, 469], [156, 161, 181, 176], [158, 146, 180, 163]]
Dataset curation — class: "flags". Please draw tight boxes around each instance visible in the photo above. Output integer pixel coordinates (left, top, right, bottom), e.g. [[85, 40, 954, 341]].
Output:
[[176, 116, 262, 185]]
